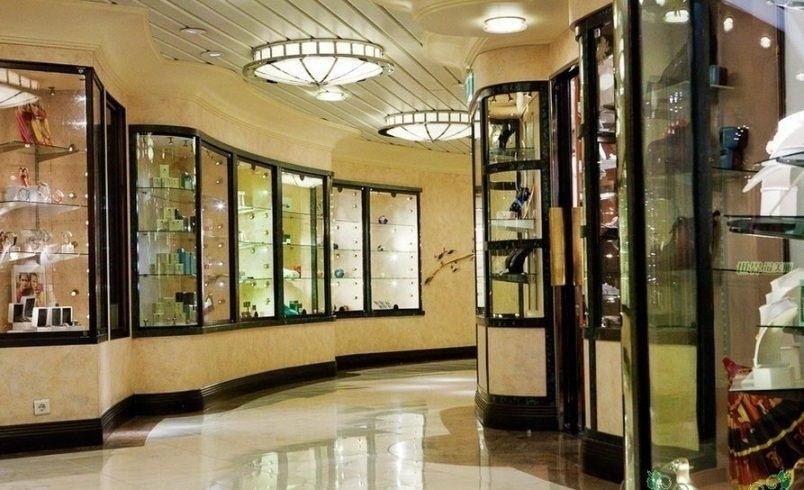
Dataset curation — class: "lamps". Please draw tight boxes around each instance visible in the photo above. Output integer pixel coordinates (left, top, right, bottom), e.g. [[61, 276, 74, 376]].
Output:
[[378, 107, 475, 147], [240, 35, 396, 87]]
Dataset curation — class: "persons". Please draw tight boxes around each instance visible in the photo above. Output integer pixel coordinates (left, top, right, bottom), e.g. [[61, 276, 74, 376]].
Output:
[[16, 271, 45, 308], [14, 98, 55, 147]]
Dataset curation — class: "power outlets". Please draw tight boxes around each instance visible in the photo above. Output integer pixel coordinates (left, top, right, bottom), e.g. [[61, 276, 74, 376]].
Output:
[[33, 398, 51, 417]]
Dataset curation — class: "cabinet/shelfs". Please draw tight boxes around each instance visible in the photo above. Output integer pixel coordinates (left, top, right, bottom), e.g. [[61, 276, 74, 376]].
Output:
[[130, 123, 335, 337], [329, 179, 424, 319], [468, 0, 783, 345], [0, 58, 131, 345]]
